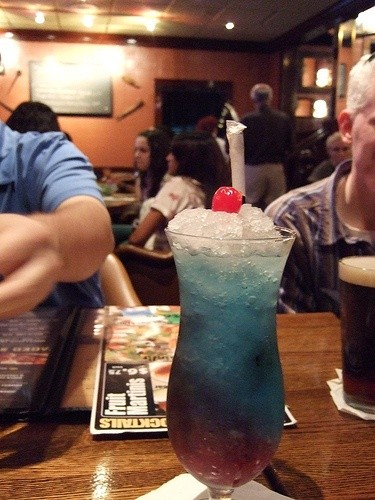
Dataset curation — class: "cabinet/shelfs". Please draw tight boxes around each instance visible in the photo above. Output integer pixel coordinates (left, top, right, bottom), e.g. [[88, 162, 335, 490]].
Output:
[[290, 44, 338, 120]]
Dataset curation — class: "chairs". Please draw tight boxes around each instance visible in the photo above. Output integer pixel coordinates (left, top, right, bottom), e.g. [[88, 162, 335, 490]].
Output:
[[117, 245, 180, 307]]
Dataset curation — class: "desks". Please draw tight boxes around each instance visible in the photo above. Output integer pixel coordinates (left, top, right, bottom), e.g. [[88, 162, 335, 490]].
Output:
[[0, 311, 375, 500]]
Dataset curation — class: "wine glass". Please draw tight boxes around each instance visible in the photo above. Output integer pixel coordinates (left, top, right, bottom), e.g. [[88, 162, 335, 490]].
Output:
[[165, 225, 296, 499]]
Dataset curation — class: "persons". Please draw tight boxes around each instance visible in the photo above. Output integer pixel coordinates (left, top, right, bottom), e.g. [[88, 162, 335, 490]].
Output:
[[308, 133, 355, 182], [5, 101, 60, 133], [103, 129, 166, 204], [265, 51, 375, 313], [240, 84, 294, 206], [116, 132, 229, 253], [0, 121, 115, 321], [308, 116, 341, 166]]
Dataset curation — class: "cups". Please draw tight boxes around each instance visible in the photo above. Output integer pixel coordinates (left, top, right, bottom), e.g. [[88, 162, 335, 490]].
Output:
[[337, 255, 375, 413]]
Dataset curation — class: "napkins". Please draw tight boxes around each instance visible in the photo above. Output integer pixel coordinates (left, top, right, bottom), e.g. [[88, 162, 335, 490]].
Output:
[[327, 368, 375, 421], [138, 471, 298, 500]]
